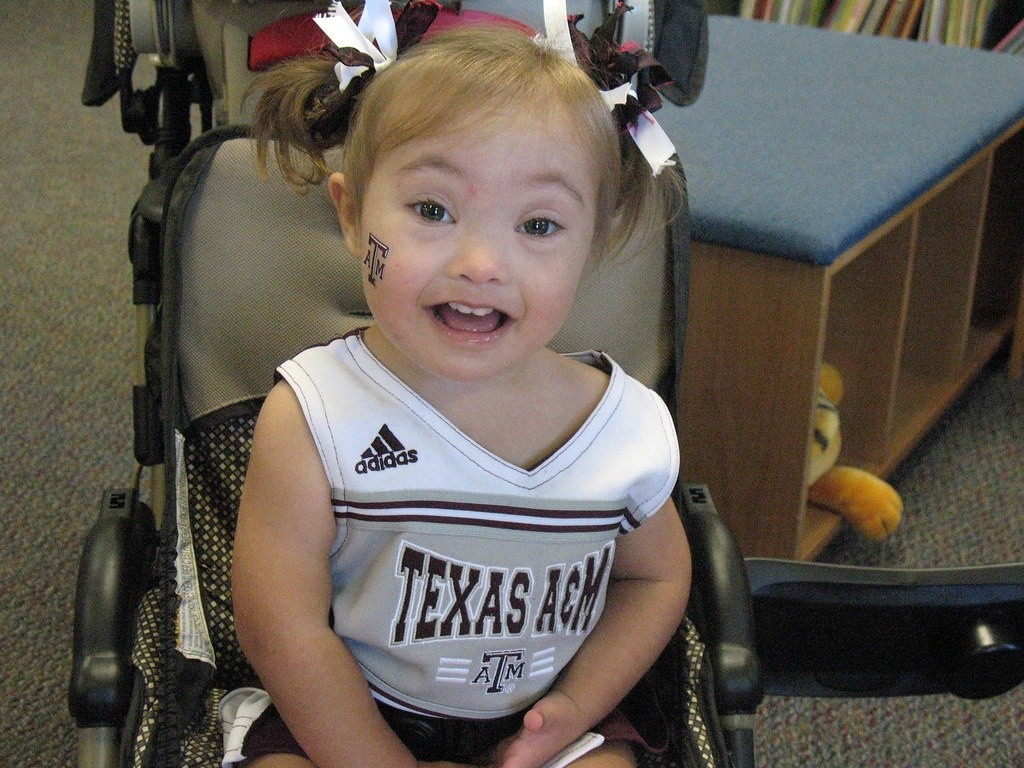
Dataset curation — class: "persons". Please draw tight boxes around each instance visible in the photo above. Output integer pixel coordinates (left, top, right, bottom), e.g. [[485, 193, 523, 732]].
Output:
[[219, 0, 693, 768]]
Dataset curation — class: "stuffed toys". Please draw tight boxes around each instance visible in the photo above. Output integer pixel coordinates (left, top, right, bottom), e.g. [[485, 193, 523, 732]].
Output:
[[804, 362, 904, 540]]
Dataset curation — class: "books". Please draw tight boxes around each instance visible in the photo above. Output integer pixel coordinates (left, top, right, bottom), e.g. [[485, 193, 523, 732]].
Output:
[[742, 0, 1024, 62]]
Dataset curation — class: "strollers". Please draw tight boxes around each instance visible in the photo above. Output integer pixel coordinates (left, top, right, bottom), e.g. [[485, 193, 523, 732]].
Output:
[[62, 1, 768, 768]]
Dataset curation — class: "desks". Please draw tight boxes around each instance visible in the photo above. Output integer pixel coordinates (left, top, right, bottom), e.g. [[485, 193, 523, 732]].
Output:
[[657, 16, 1024, 560]]
